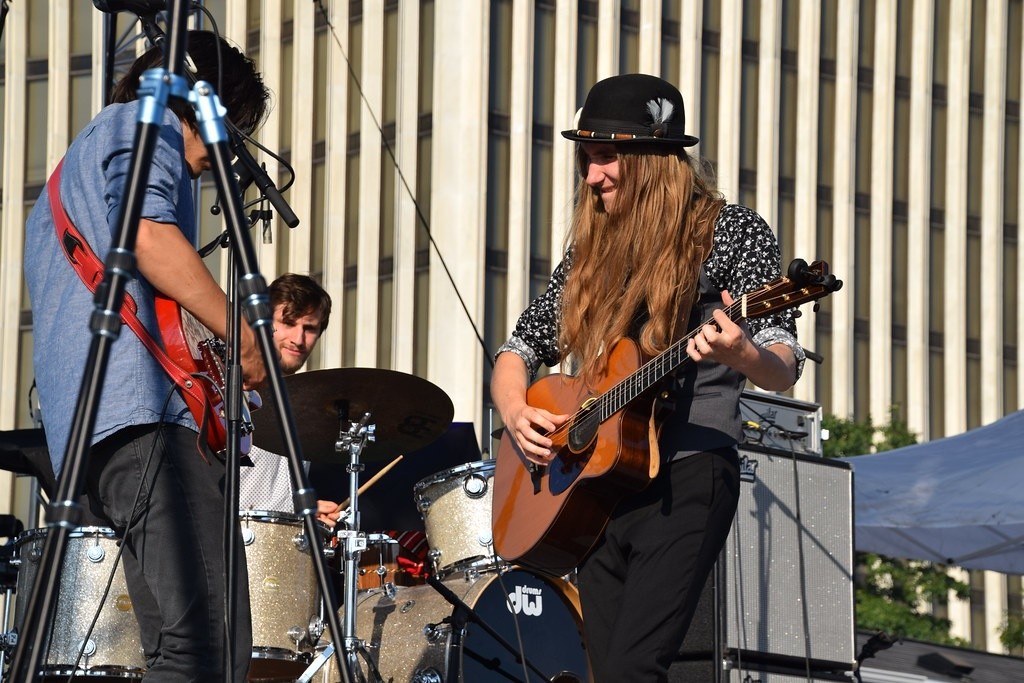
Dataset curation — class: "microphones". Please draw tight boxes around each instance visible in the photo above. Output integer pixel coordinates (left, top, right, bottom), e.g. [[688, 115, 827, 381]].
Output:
[[260, 162, 272, 244], [93, 0, 202, 15]]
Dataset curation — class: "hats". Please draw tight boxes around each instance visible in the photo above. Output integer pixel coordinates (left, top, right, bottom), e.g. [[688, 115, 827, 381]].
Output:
[[561, 73, 702, 147]]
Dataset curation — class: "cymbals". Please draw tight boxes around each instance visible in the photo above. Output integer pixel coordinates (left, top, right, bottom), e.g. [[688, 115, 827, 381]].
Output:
[[0, 427, 52, 473], [252, 367, 455, 463]]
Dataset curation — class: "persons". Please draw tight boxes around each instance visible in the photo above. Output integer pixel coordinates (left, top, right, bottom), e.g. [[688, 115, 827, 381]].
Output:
[[490, 75, 805, 682], [239, 274, 340, 527], [27, 29, 282, 683]]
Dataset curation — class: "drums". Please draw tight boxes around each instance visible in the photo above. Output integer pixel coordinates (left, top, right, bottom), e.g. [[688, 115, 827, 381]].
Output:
[[10, 525, 148, 683], [236, 509, 334, 683], [309, 563, 595, 683], [412, 458, 513, 580]]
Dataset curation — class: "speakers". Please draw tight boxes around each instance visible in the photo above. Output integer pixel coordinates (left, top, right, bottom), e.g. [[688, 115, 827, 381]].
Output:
[[662, 442, 858, 683]]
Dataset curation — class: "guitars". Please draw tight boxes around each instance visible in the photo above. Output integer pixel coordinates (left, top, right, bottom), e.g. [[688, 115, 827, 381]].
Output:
[[491, 258, 843, 577]]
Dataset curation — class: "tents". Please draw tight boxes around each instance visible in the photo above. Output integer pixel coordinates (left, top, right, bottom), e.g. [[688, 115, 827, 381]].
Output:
[[834, 409, 1024, 574]]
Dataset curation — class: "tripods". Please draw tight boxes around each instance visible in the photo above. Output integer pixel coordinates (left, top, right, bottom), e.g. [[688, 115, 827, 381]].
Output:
[[296, 413, 386, 683]]
